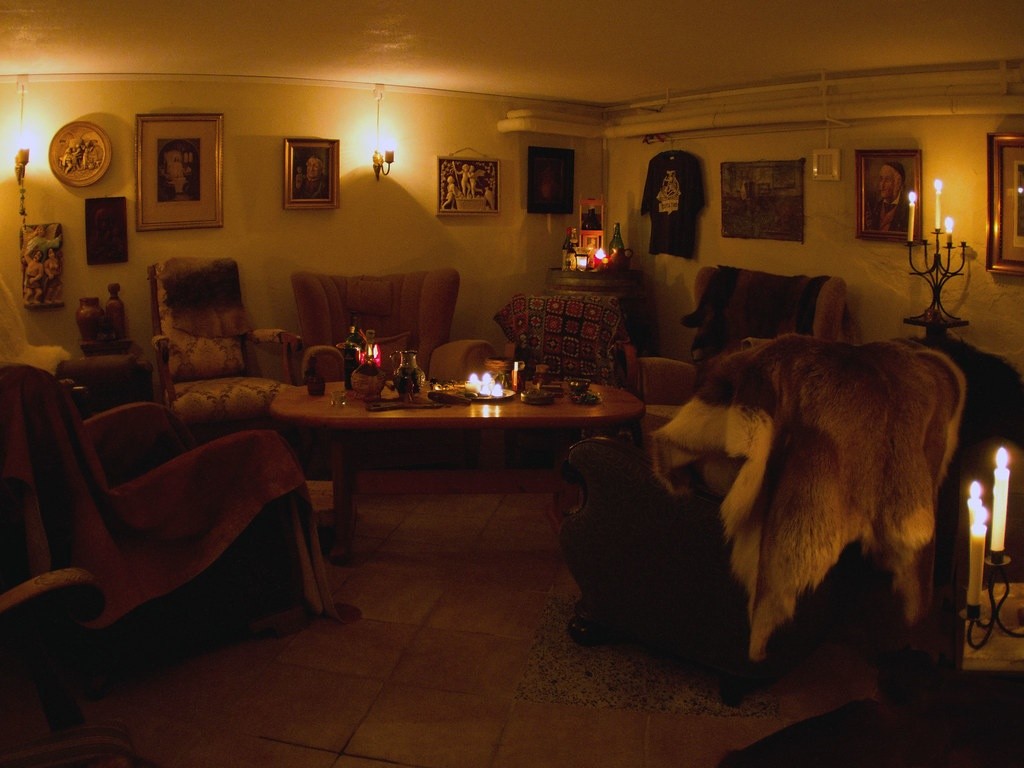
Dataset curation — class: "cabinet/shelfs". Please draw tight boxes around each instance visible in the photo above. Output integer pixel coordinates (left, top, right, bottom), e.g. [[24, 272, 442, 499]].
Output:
[[55, 345, 151, 414]]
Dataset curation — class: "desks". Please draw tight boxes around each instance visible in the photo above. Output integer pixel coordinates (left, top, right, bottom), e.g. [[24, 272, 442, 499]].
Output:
[[547, 267, 642, 355], [270, 381, 646, 562]]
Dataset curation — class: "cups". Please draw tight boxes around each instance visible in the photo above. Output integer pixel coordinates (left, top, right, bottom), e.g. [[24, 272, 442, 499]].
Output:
[[576, 257, 587, 272], [564, 375, 592, 391]]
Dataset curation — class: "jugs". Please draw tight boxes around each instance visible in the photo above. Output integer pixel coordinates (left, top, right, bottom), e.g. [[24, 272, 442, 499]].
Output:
[[389, 350, 426, 401]]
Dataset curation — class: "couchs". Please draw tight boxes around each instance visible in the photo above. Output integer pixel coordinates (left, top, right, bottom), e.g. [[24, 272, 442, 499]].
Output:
[[1, 362, 360, 632], [632, 265, 846, 442], [564, 336, 964, 705], [293, 271, 501, 473]]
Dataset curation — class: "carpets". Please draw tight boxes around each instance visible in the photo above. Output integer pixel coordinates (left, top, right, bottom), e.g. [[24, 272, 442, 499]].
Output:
[[516, 593, 779, 717]]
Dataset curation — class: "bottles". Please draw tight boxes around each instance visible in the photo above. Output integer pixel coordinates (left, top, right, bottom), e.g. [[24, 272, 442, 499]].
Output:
[[600, 222, 626, 272], [308, 377, 326, 396], [532, 366, 552, 386], [562, 227, 578, 272], [511, 361, 525, 393], [345, 313, 386, 395], [582, 206, 600, 230], [75, 280, 127, 343]]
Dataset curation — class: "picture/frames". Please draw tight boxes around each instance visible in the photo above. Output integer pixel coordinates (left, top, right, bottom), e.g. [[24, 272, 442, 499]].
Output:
[[284, 138, 340, 209], [133, 112, 224, 231], [986, 132, 1024, 276], [855, 149, 922, 245], [527, 146, 574, 214]]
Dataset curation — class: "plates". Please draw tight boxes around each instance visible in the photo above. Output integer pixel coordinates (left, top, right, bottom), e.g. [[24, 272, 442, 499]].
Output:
[[444, 384, 516, 402]]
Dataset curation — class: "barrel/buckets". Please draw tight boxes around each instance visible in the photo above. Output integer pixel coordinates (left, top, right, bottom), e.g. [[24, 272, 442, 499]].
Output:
[[484, 356, 515, 389]]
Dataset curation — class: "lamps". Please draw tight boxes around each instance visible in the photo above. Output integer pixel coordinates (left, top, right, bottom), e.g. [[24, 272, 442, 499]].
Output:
[[374, 149, 393, 182]]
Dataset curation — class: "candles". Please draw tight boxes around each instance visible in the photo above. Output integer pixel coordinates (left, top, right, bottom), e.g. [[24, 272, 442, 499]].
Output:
[[908, 202, 915, 242], [946, 229, 952, 243], [936, 192, 941, 229], [968, 468, 1009, 606]]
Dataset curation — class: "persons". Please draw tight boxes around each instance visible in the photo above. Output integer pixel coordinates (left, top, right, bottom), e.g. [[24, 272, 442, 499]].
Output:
[[24, 249, 44, 305], [43, 248, 60, 305]]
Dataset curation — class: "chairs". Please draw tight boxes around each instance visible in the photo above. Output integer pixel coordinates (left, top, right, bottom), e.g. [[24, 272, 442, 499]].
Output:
[[149, 258, 297, 436], [493, 293, 645, 469]]
